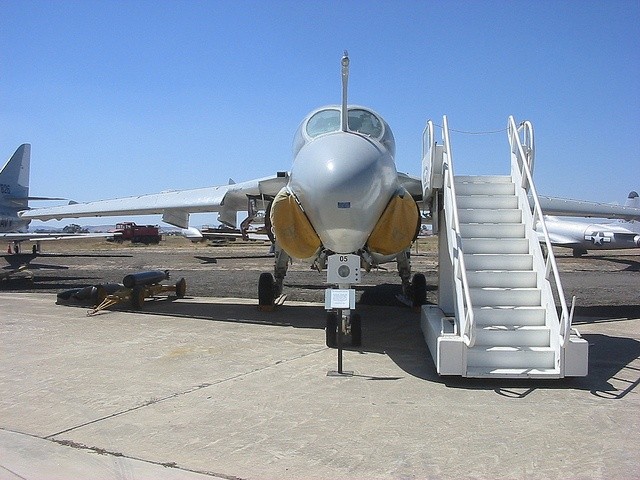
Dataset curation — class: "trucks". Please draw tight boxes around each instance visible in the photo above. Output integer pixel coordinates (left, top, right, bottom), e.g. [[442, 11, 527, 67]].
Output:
[[106, 221, 161, 245]]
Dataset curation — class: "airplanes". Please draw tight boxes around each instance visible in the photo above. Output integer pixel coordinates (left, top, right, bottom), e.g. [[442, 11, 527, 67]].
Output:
[[0, 143, 123, 254], [17, 51, 640, 347], [535, 191, 640, 258]]
